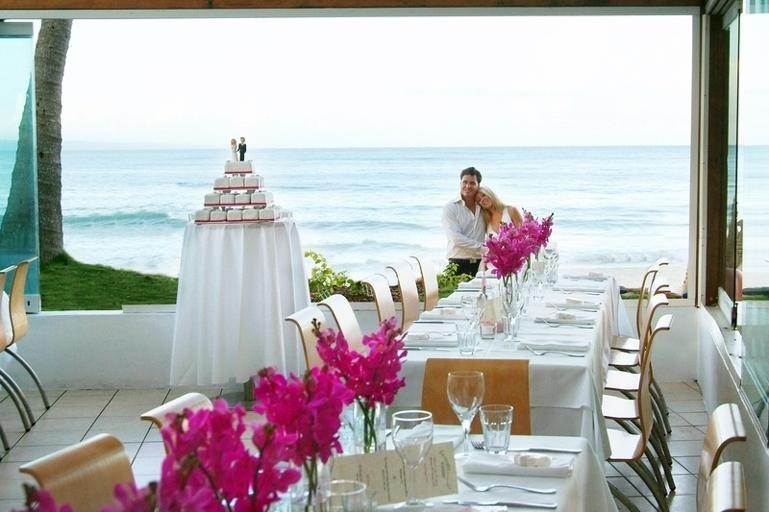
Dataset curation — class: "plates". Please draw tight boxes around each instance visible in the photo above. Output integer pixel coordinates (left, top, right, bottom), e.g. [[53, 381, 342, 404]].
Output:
[[454, 447, 578, 481]]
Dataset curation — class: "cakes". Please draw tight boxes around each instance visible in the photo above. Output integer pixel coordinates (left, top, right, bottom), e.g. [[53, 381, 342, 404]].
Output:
[[196, 136, 280, 223]]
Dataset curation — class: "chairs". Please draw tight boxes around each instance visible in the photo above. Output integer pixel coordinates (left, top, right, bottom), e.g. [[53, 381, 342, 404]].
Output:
[[284, 252, 438, 436], [0, 260, 32, 461], [695, 399, 749, 511], [602, 258, 677, 511], [1, 255, 54, 432]]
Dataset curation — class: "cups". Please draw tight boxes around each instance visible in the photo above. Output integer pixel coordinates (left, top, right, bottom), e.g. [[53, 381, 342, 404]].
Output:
[[263, 459, 305, 512], [474, 403, 514, 459], [312, 476, 371, 512]]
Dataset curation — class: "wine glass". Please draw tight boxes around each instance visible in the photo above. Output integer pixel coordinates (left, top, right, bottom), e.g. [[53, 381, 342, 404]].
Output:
[[391, 408, 438, 511], [389, 244, 613, 360], [441, 371, 485, 459]]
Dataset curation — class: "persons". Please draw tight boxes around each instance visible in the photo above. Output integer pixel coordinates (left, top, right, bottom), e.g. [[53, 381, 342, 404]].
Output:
[[227, 138, 239, 162], [235, 136, 247, 161], [475, 186, 524, 245], [442, 166, 491, 284]]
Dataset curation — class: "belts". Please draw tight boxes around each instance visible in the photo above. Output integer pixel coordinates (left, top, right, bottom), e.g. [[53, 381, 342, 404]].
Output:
[[469, 258, 477, 264]]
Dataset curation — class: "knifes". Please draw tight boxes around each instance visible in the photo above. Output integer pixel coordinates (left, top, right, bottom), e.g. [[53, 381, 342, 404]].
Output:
[[432, 492, 560, 508], [499, 442, 585, 457]]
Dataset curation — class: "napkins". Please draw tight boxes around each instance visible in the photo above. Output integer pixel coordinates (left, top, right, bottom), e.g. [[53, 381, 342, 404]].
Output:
[[511, 271, 608, 353], [458, 453, 574, 477], [404, 271, 500, 349]]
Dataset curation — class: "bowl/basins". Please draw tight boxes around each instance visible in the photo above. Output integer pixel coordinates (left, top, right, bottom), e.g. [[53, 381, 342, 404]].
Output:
[[388, 422, 466, 457]]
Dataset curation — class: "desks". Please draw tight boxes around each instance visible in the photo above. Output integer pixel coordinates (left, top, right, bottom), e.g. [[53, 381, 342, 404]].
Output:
[[378, 270, 616, 464], [280, 432, 591, 511], [168, 217, 310, 402]]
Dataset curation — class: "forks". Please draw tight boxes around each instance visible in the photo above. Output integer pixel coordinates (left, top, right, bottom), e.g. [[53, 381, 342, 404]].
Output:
[[455, 472, 556, 495], [467, 437, 486, 452]]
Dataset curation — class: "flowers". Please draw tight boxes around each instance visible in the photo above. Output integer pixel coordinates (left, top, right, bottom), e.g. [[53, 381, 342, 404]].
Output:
[[20, 313, 406, 511], [483, 208, 554, 321]]
